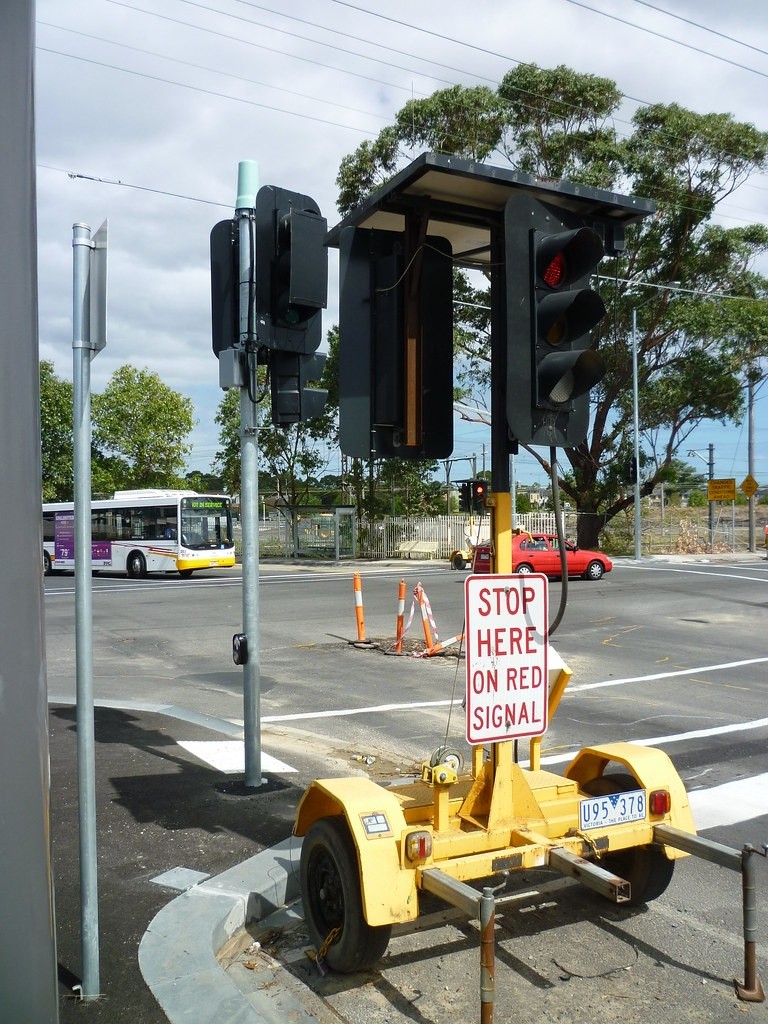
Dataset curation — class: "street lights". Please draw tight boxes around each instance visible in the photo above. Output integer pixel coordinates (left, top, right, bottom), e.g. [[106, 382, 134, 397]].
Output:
[[657, 453, 665, 534], [632, 281, 682, 560]]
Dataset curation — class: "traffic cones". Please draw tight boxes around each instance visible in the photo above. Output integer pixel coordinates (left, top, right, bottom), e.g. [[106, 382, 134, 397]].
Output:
[[385, 578, 412, 655], [350, 573, 371, 644], [413, 582, 466, 657]]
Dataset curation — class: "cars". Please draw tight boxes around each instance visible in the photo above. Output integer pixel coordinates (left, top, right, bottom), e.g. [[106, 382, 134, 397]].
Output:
[[472, 533, 612, 580]]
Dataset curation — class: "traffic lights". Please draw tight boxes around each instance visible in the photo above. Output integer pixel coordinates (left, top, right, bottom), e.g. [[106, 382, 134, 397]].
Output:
[[272, 351, 328, 424], [458, 487, 468, 512], [474, 481, 487, 511], [529, 226, 608, 411]]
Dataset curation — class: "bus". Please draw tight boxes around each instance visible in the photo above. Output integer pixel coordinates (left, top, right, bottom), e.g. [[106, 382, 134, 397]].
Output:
[[43, 489, 239, 578]]
